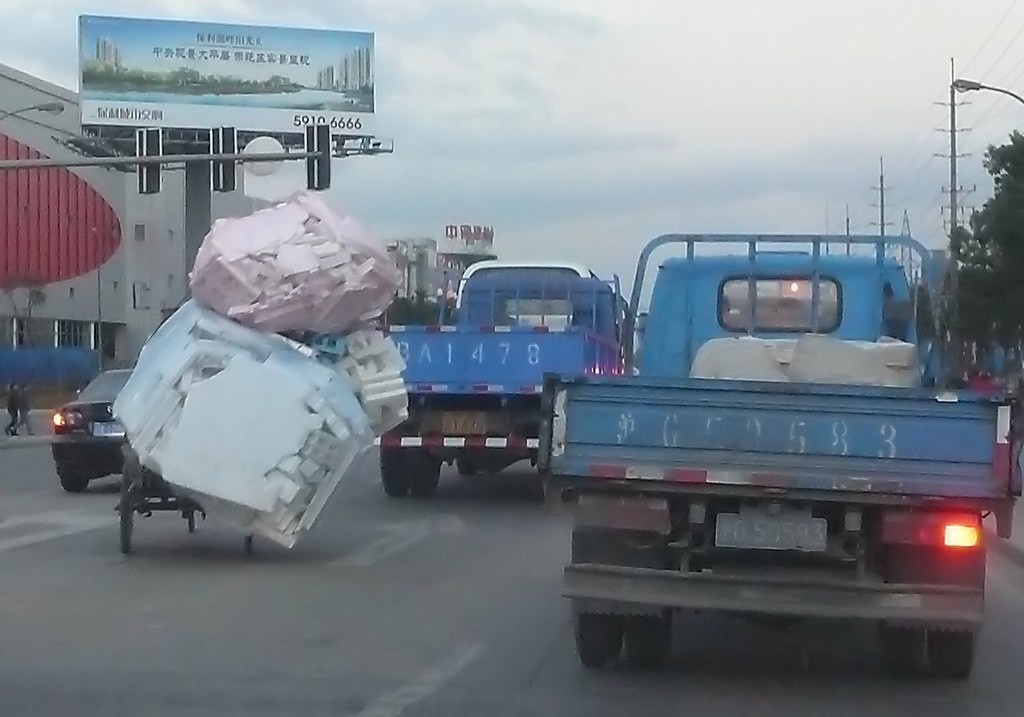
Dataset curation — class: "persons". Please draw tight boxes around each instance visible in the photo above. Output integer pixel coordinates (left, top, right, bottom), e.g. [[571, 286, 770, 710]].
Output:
[[4, 382, 35, 438]]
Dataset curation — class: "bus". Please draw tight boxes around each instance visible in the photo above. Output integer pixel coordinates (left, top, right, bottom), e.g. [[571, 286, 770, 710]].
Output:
[[454, 260, 638, 375]]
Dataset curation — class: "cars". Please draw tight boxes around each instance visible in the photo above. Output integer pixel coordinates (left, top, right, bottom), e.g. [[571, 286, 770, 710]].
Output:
[[51, 369, 135, 492]]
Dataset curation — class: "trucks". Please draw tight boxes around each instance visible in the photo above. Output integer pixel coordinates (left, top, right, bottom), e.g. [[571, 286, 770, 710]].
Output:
[[535, 233, 1013, 679], [377, 276, 627, 497]]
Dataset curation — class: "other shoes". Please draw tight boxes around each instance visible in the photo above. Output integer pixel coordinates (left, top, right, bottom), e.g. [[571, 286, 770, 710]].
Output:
[[13, 433, 19, 436], [27, 432, 35, 435], [5, 428, 9, 436], [10, 427, 14, 436]]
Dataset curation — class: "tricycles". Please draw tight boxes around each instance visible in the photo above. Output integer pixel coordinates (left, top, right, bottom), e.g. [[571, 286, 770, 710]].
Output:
[[113, 441, 251, 556]]
